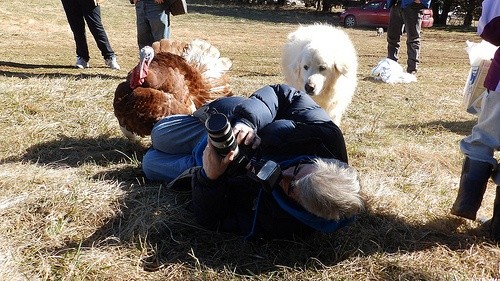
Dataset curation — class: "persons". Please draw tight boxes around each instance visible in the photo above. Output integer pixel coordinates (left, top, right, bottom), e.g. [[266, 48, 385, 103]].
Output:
[[59, 0, 121, 70], [142, 83, 367, 245], [449, 0, 500, 241], [387, 0, 432, 74], [128, 0, 188, 56]]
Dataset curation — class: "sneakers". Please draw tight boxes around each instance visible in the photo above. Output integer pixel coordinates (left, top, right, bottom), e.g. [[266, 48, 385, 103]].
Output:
[[106, 57, 120, 69], [75, 57, 89, 69]]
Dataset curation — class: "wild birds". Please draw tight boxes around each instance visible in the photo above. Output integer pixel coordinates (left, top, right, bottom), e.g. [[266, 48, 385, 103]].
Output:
[[114, 38, 232, 148]]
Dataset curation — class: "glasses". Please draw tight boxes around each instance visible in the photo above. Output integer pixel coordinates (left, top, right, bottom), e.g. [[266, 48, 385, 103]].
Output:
[[287, 159, 311, 200]]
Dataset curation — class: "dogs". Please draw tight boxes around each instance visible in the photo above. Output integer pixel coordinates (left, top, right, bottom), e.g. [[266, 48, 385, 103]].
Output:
[[370, 57, 417, 84], [280, 20, 358, 129]]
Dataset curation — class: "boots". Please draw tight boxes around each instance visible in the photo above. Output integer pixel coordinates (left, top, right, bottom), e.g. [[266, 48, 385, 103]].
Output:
[[450, 157, 493, 220], [487, 186, 500, 238]]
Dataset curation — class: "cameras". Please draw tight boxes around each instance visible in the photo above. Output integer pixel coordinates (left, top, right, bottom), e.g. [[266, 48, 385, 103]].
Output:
[[204, 112, 282, 189]]
